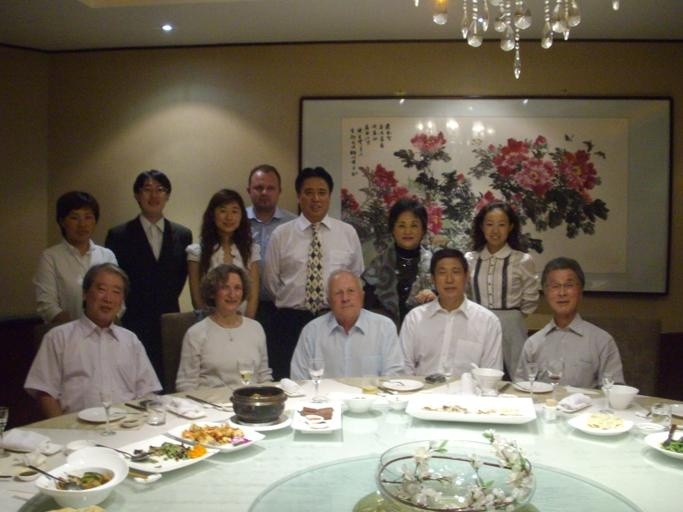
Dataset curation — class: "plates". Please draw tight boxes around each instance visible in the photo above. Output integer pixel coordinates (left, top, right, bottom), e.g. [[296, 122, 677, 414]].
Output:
[[224, 413, 293, 431], [381, 379, 424, 392], [77, 406, 126, 422], [19, 471, 38, 483], [290, 401, 344, 434], [642, 430, 682, 462], [663, 403, 682, 418], [566, 412, 635, 436], [276, 385, 306, 398], [512, 381, 552, 393], [161, 394, 208, 420], [116, 435, 220, 475], [120, 417, 141, 427], [166, 421, 268, 456], [405, 393, 538, 425]]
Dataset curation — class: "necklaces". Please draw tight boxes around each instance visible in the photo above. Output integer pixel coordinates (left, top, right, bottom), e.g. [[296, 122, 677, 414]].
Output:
[[400, 256, 415, 270], [212, 316, 239, 342]]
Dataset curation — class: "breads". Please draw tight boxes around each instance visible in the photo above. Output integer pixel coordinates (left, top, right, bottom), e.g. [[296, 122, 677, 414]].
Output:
[[363, 385, 379, 394]]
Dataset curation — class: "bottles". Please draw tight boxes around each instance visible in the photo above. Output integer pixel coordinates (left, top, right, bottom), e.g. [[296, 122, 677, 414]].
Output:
[[542, 399, 557, 422]]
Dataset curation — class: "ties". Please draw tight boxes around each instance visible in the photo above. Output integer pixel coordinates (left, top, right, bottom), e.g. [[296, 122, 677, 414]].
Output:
[[305, 222, 329, 315]]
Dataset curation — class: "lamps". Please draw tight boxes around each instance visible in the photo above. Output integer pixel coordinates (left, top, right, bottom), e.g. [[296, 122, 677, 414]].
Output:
[[411, 1, 623, 79]]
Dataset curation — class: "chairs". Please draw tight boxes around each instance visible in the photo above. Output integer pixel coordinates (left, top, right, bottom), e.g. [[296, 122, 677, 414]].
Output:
[[0, 312, 70, 428], [589, 309, 663, 402], [157, 310, 242, 397]]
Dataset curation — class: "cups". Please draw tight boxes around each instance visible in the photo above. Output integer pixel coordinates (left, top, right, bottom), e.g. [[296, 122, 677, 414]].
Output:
[[360, 364, 378, 394], [147, 400, 168, 425], [650, 402, 672, 429], [236, 358, 256, 385], [0, 406, 8, 436]]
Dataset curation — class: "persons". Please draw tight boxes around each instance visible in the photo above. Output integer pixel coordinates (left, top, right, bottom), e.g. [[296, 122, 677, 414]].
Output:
[[175, 264, 273, 391], [516, 257, 624, 389], [24, 263, 164, 417], [464, 201, 540, 381], [360, 197, 439, 335], [291, 270, 405, 382], [106, 169, 193, 368], [242, 164, 299, 323], [263, 167, 365, 380], [400, 249, 504, 373], [185, 189, 261, 319], [32, 190, 127, 326]]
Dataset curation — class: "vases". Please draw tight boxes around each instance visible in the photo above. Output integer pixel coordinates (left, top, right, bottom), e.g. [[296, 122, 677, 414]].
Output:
[[375, 439, 540, 512]]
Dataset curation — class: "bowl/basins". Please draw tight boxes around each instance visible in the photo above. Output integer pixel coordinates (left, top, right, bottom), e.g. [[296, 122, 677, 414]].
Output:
[[388, 398, 407, 411], [344, 396, 376, 412], [228, 386, 289, 422], [66, 439, 96, 454], [471, 367, 505, 390], [601, 384, 640, 409], [34, 446, 130, 509]]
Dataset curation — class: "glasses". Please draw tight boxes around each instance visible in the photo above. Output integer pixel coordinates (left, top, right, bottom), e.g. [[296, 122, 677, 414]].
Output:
[[549, 281, 579, 291], [142, 186, 168, 193]]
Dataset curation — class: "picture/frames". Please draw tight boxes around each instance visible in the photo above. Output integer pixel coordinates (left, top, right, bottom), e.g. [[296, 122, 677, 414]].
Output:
[[296, 93, 673, 300]]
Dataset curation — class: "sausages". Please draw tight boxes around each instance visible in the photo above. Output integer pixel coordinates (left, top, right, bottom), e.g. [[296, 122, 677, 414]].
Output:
[[300, 407, 334, 419]]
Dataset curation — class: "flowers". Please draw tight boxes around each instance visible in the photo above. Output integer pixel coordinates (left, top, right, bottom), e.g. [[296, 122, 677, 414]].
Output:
[[339, 123, 610, 260], [381, 424, 534, 512]]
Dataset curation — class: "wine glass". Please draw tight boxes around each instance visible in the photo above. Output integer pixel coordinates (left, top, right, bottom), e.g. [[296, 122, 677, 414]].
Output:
[[99, 388, 114, 434], [546, 357, 564, 398], [308, 357, 327, 404], [597, 364, 618, 411], [440, 360, 452, 391], [526, 362, 539, 396]]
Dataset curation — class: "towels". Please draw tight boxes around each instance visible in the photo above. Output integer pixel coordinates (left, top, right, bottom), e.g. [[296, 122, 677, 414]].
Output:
[[0, 426, 59, 457], [276, 376, 306, 398], [166, 397, 206, 419], [556, 391, 593, 414]]
